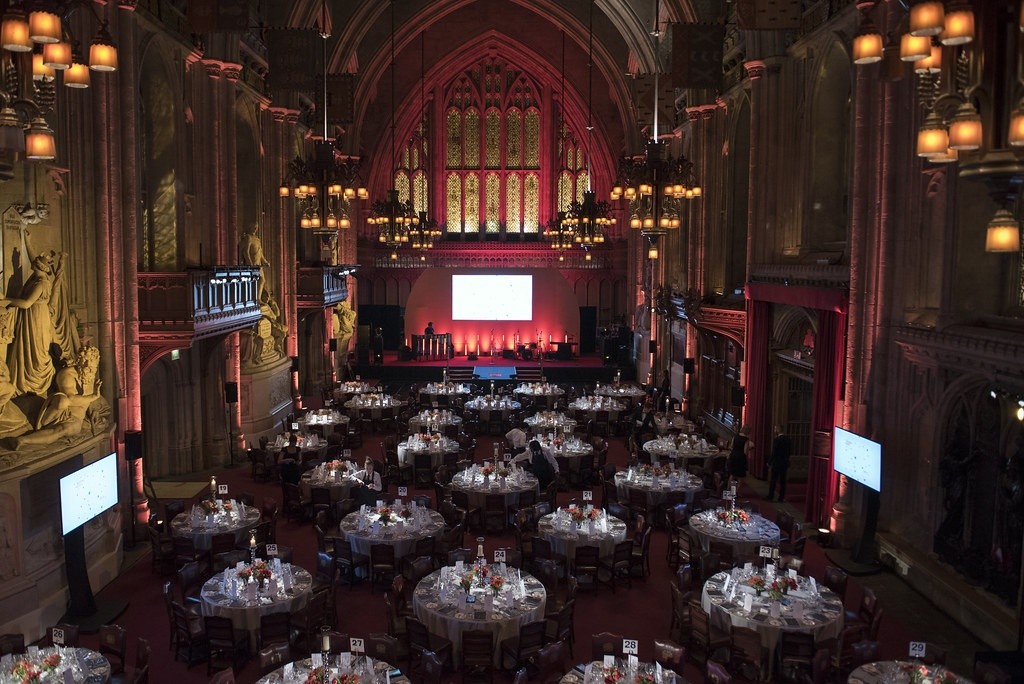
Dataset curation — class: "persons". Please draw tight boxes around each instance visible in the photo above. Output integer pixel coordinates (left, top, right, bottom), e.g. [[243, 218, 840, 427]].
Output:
[[505, 422, 529, 454], [239, 221, 271, 305], [510, 444, 559, 493], [425, 322, 434, 334], [721, 424, 754, 492], [767, 425, 793, 502], [0, 227, 110, 450], [632, 394, 654, 436], [278, 435, 303, 484], [662, 369, 671, 397], [435, 465, 453, 486], [348, 456, 382, 507]]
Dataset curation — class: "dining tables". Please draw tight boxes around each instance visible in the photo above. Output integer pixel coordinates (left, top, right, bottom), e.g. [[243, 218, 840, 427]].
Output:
[[463, 397, 521, 423], [266, 433, 328, 464], [301, 461, 365, 511], [540, 506, 627, 562], [418, 384, 471, 402], [689, 508, 780, 565], [701, 566, 845, 656], [568, 394, 627, 424], [643, 438, 720, 469], [200, 562, 313, 655], [256, 654, 411, 683], [513, 384, 565, 407], [523, 411, 577, 435], [452, 461, 539, 529], [409, 409, 463, 436], [412, 562, 547, 670], [343, 393, 401, 426], [340, 381, 378, 396], [169, 497, 261, 551], [526, 433, 593, 459], [593, 384, 647, 410], [641, 412, 698, 432], [847, 661, 974, 684], [340, 504, 446, 578], [397, 433, 459, 471], [560, 659, 684, 684], [1, 646, 111, 683], [295, 409, 351, 441], [614, 466, 704, 519]]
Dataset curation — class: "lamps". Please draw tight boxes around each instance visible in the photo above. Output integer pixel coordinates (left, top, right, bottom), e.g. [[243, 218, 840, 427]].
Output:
[[367, 0, 420, 262], [566, 1, 617, 262], [1, 0, 116, 159], [851, 0, 1024, 251], [610, 0, 702, 259], [410, 31, 443, 262], [541, 31, 567, 272], [278, 0, 369, 245]]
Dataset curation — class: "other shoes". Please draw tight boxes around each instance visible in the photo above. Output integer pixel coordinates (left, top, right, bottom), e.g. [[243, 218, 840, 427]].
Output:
[[762, 496, 773, 502], [773, 500, 783, 503], [737, 494, 744, 498]]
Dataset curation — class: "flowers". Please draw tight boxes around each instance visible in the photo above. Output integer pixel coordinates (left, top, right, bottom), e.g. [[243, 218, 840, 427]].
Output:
[[479, 464, 494, 478], [460, 573, 474, 594], [473, 565, 490, 589], [497, 467, 509, 477], [902, 665, 929, 683], [221, 500, 233, 513], [304, 666, 333, 683], [420, 434, 431, 447], [237, 569, 251, 586], [9, 659, 42, 684], [768, 577, 798, 594], [603, 668, 623, 684], [399, 507, 412, 522], [489, 576, 505, 598], [200, 499, 220, 515], [933, 671, 957, 684], [378, 507, 391, 526], [249, 567, 272, 588], [737, 511, 750, 524], [715, 511, 731, 525], [747, 575, 766, 596], [42, 654, 62, 674], [636, 673, 656, 684], [332, 673, 360, 683]]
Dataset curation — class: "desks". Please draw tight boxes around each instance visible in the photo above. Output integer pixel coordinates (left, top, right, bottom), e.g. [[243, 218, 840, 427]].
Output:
[[412, 333, 454, 362], [550, 342, 578, 360]]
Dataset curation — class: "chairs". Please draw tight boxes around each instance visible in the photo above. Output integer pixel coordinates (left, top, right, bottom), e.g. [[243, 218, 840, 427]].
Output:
[[1, 380, 974, 684]]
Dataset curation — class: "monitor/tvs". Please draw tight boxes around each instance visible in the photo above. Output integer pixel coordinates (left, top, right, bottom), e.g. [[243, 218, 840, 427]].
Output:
[[58, 451, 120, 537], [832, 426, 882, 491]]
